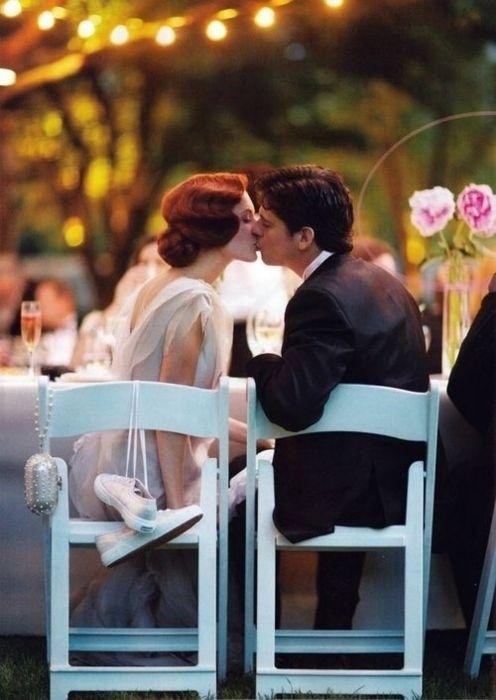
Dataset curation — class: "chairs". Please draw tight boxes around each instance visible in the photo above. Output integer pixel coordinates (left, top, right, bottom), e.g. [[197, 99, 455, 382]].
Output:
[[33, 372, 231, 700], [243, 376, 440, 699]]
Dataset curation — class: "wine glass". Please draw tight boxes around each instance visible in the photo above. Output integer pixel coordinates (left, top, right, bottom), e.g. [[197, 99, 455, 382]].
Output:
[[21, 302, 44, 378]]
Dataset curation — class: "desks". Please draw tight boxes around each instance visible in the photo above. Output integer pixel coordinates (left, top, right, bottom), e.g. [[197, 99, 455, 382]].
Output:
[[3, 367, 496, 642]]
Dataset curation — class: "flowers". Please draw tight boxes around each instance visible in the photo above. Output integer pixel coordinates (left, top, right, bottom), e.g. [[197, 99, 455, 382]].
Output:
[[409, 181, 495, 356]]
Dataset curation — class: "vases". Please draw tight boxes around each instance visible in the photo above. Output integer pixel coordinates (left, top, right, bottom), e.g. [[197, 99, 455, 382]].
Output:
[[437, 252, 471, 377]]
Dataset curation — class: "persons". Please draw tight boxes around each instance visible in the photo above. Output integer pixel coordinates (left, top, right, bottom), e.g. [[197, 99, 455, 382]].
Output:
[[227, 165, 430, 666], [70, 236, 168, 371], [68, 173, 276, 669], [352, 235, 399, 279], [1, 273, 77, 369], [441, 273, 495, 631]]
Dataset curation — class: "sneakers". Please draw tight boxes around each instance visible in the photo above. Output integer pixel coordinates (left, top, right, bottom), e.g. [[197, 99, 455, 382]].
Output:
[[94, 504, 204, 569], [93, 473, 158, 533]]
